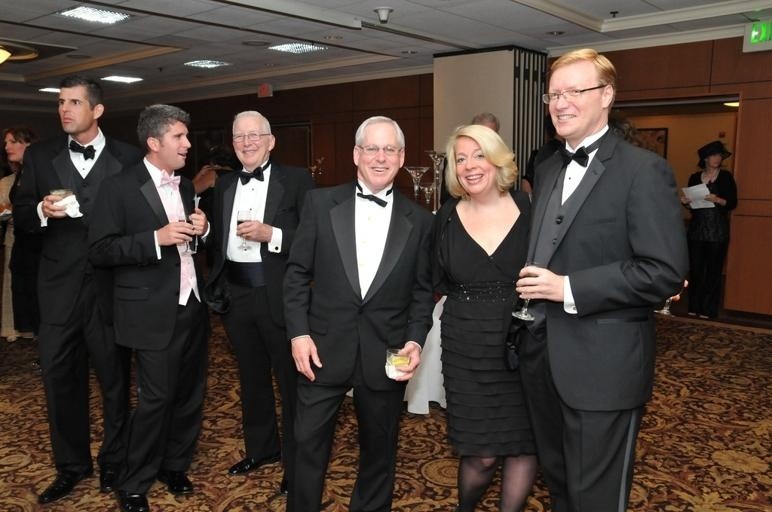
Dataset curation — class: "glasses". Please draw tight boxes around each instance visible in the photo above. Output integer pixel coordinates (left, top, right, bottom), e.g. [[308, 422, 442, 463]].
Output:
[[542, 85, 607, 104], [358, 145, 403, 154], [233, 132, 270, 142]]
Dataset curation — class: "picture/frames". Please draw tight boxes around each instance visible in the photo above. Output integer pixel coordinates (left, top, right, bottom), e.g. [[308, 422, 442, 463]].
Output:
[[628, 128, 668, 161]]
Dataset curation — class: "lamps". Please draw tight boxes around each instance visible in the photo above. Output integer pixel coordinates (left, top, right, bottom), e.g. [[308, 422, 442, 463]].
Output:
[[0, 45, 13, 64]]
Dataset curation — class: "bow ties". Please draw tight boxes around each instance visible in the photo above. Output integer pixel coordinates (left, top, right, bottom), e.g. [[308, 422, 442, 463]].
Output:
[[160, 169, 181, 190], [560, 146, 588, 167], [239, 167, 264, 185], [70, 140, 95, 159], [357, 193, 387, 207]]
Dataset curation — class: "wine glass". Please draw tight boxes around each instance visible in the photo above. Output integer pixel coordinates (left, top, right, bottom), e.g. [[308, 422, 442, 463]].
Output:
[[235, 209, 255, 253], [512, 261, 546, 321], [174, 215, 196, 258]]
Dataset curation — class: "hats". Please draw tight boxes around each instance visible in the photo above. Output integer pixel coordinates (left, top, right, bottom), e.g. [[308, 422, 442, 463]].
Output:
[[698, 141, 732, 161]]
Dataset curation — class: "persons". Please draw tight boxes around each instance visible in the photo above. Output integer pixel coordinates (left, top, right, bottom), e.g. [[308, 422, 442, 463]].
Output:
[[1, 127, 38, 341], [679, 139, 739, 321], [86, 101, 214, 510], [203, 108, 317, 495], [8, 76, 145, 501], [438, 111, 533, 206], [280, 114, 436, 511], [517, 50, 689, 510], [399, 123, 691, 511]]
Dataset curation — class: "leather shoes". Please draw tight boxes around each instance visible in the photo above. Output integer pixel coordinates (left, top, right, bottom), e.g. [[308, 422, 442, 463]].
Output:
[[280, 469, 295, 494], [228, 458, 279, 475], [38, 470, 194, 512]]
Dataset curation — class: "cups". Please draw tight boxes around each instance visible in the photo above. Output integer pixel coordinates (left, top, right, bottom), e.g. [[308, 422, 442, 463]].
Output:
[[385, 347, 411, 382], [48, 188, 73, 204]]
